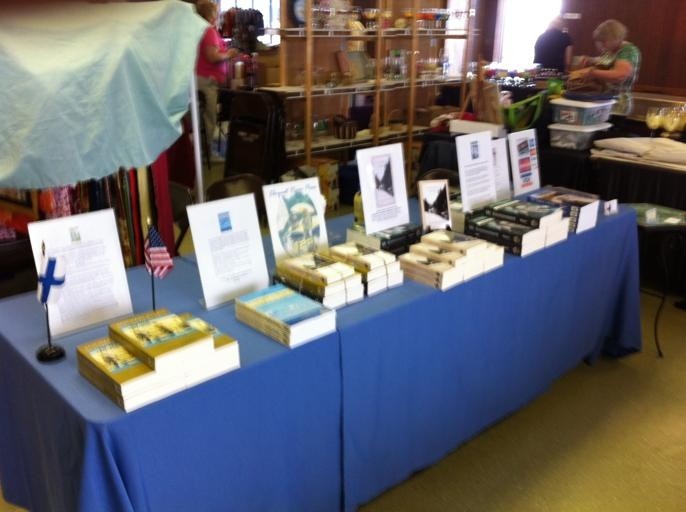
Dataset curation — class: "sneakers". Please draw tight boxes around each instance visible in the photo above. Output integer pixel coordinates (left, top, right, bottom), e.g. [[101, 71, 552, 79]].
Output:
[[206, 138, 226, 162]]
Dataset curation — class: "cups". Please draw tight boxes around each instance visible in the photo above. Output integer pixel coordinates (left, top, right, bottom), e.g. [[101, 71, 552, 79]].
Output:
[[388, 118, 406, 131], [285, 115, 330, 150], [296, 65, 352, 89]]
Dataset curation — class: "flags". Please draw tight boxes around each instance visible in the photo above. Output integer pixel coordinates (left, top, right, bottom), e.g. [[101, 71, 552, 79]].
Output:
[[140, 223, 173, 280]]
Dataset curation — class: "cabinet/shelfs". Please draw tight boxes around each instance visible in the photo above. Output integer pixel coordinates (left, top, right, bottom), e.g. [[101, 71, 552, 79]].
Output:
[[254, 0, 481, 220]]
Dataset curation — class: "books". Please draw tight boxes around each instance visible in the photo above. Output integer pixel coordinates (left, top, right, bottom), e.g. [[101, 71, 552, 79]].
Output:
[[515, 185, 599, 234], [316, 240, 403, 296], [450, 196, 469, 233], [345, 222, 422, 261], [234, 283, 336, 348], [76, 303, 245, 413], [467, 198, 568, 258], [397, 229, 504, 291], [275, 253, 365, 310]]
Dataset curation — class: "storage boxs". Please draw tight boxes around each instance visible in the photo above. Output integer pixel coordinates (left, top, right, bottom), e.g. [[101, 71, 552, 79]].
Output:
[[547, 124, 613, 150], [549, 98, 617, 126]]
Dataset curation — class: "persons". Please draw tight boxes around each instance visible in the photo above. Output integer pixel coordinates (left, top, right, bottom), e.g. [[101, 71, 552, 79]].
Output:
[[194, 1, 239, 164], [533, 18, 574, 74], [571, 21, 642, 118]]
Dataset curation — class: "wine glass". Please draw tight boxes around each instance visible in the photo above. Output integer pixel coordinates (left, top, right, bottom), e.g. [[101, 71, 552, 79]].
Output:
[[467, 57, 542, 88], [370, 48, 451, 88], [645, 104, 686, 139], [310, 5, 471, 35]]
